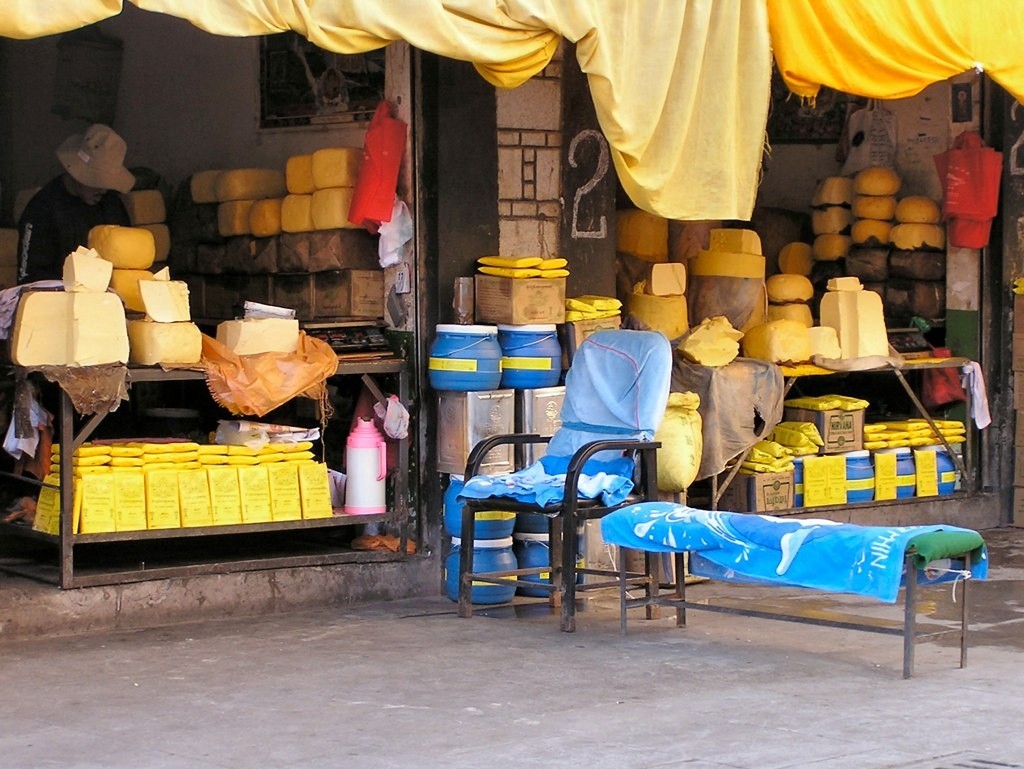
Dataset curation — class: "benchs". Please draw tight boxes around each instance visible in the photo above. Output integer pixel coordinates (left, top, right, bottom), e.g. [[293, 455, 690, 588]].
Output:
[[602, 501, 987, 679]]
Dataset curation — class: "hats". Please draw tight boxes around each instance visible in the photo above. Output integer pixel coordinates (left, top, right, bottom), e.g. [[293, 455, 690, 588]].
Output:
[[57, 123, 136, 196]]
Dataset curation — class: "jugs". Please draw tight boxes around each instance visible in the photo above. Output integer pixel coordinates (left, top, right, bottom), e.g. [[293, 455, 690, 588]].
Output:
[[343, 415, 389, 515]]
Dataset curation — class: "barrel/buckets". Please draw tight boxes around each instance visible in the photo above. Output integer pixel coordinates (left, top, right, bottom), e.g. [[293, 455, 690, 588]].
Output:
[[516, 509, 590, 536], [425, 321, 503, 392], [441, 472, 518, 538], [793, 445, 956, 508], [493, 324, 564, 390], [510, 529, 579, 597], [440, 533, 520, 604]]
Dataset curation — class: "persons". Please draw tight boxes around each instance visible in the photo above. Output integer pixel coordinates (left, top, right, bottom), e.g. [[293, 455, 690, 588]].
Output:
[[19, 121, 136, 282]]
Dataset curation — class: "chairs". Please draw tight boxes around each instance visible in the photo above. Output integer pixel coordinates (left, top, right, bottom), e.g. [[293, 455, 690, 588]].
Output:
[[459, 330, 672, 632]]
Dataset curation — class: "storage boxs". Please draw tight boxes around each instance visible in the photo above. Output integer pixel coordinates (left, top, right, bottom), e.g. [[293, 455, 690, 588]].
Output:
[[719, 472, 794, 512], [556, 315, 621, 368], [475, 275, 566, 324], [623, 490, 711, 585], [169, 203, 387, 323], [786, 408, 863, 452]]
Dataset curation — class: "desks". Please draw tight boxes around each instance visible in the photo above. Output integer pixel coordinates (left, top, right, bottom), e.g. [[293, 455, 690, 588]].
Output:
[[677, 357, 972, 510], [0, 359, 408, 590]]
